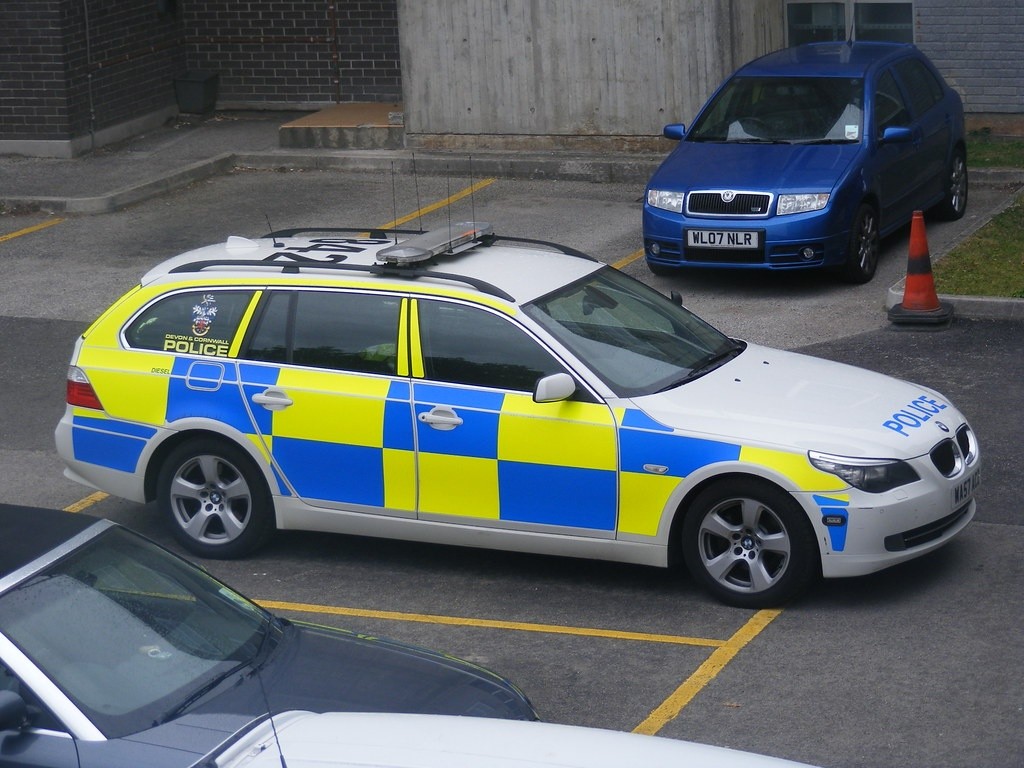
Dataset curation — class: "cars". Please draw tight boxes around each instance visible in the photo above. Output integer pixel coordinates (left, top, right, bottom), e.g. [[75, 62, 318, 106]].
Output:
[[642, 42, 968, 286], [0, 504, 542, 768], [54, 226, 979, 607]]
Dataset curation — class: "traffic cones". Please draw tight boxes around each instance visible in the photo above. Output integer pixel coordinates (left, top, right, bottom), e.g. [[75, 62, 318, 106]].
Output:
[[887, 210, 955, 331]]
